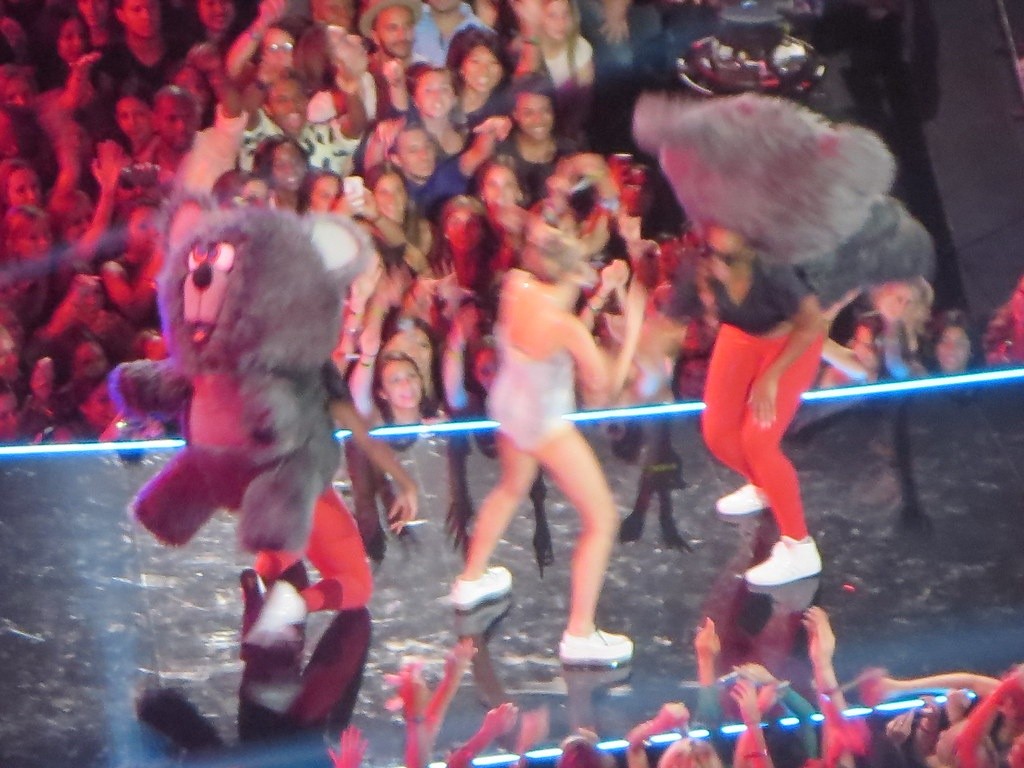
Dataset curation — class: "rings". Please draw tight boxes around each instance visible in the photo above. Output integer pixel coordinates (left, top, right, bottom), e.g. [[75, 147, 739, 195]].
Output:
[[900, 721, 904, 724]]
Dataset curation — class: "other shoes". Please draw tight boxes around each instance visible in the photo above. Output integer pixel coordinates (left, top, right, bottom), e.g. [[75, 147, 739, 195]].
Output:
[[246, 581, 307, 646]]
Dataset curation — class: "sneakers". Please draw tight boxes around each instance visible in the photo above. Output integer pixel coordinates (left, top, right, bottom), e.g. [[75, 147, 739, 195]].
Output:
[[455, 595, 513, 636], [715, 482, 771, 514], [451, 565, 511, 611], [562, 667, 632, 695], [747, 579, 825, 609], [745, 536, 822, 586], [558, 625, 634, 665]]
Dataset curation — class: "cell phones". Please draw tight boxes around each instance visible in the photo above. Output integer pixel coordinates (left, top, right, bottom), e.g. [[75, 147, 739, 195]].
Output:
[[717, 672, 739, 691], [344, 176, 364, 214]]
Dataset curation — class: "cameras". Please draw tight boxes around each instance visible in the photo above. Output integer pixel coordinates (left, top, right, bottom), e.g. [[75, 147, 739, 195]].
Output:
[[120, 163, 161, 191]]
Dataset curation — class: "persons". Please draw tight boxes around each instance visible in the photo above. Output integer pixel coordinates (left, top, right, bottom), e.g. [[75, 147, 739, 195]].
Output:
[[241, 358, 419, 654], [327, 605, 1024, 768], [0, 0, 1024, 447], [450, 233, 646, 663], [697, 226, 827, 586]]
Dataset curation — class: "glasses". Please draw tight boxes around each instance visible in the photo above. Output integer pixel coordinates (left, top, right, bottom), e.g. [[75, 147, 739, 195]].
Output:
[[264, 41, 297, 52], [696, 243, 755, 267]]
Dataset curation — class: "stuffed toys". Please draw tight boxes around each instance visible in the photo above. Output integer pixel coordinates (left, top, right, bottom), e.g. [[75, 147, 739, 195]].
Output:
[[633, 84, 932, 304], [111, 190, 371, 549]]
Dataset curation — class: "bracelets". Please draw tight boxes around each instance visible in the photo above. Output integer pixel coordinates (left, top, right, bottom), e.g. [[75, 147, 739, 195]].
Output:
[[585, 300, 602, 315], [751, 748, 769, 758], [921, 724, 932, 734], [596, 291, 610, 302], [818, 685, 841, 702], [247, 25, 262, 40]]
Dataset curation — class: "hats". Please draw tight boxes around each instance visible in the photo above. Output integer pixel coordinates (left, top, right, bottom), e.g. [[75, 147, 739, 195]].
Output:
[[357, 0, 423, 40]]
